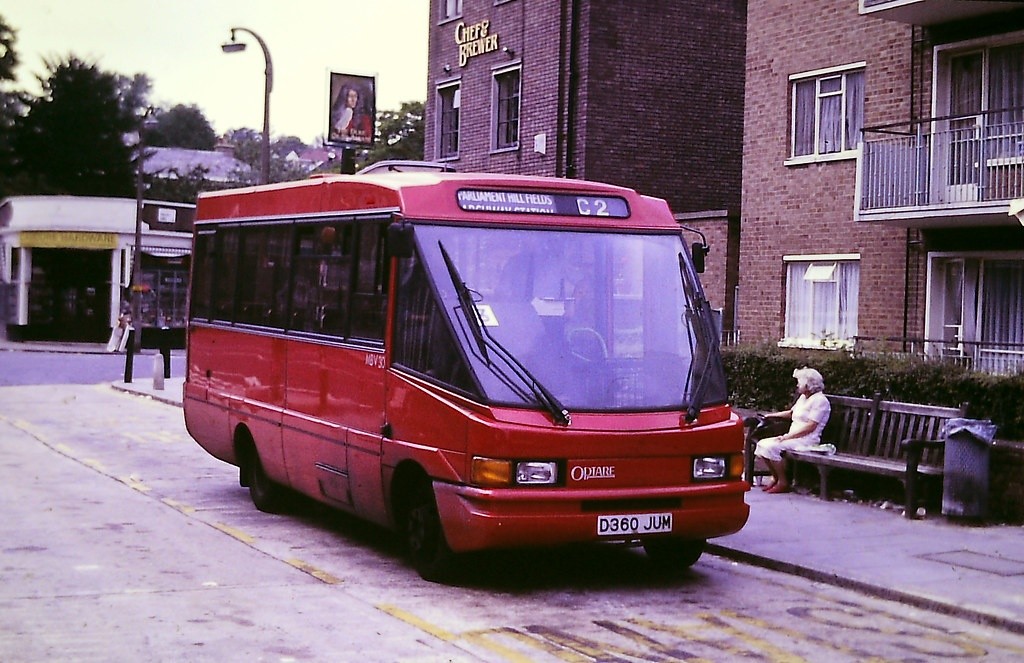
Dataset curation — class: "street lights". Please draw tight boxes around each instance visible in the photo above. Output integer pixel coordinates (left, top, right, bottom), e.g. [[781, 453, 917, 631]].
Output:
[[131, 105, 160, 354], [221, 26, 274, 185]]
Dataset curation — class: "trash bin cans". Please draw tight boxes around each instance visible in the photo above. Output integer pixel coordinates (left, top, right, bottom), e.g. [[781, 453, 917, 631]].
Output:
[[941, 417, 996, 522]]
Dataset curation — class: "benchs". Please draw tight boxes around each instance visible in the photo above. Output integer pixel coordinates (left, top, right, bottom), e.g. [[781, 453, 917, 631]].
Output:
[[740, 394, 970, 521]]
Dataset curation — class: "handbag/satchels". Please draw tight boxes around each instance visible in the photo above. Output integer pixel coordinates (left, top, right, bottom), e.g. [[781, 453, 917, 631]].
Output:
[[751, 413, 788, 444]]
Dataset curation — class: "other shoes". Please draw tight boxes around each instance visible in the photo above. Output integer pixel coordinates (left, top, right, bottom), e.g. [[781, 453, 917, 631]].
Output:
[[762, 481, 778, 491], [766, 485, 790, 493]]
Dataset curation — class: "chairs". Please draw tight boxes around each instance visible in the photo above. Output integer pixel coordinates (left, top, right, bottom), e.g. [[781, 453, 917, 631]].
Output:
[[532, 296, 564, 348]]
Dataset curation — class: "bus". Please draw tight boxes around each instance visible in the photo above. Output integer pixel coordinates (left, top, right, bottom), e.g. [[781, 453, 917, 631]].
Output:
[[181, 160, 751, 591]]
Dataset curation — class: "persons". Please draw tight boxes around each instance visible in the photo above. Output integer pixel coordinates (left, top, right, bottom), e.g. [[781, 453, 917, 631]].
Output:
[[329, 81, 367, 140], [754, 365, 831, 494]]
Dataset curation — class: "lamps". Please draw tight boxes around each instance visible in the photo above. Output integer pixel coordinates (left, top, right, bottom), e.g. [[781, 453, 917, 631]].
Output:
[[443, 64, 451, 76], [1008, 197, 1024, 226], [503, 46, 515, 59], [199, 301, 387, 340]]
